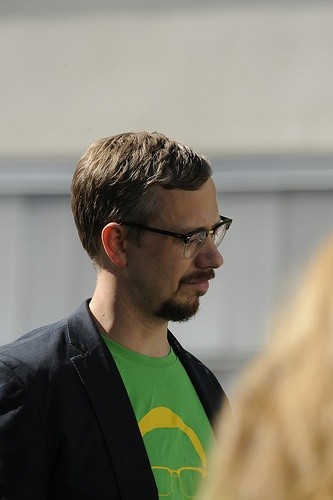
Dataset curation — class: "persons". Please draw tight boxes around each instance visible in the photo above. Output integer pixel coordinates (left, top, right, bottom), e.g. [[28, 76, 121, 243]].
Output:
[[1, 131, 234, 500]]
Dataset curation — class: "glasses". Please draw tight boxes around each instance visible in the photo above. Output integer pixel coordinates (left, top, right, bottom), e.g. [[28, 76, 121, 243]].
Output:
[[107, 217, 234, 257]]
